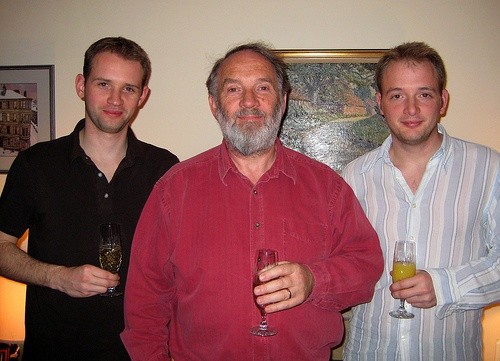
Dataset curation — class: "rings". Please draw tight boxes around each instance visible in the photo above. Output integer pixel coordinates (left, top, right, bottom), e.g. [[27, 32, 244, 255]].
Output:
[[286, 288, 291, 299]]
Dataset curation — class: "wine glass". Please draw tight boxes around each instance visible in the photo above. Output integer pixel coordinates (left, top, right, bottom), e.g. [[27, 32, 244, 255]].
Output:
[[99, 223, 122, 297], [389, 241, 417, 320], [248, 249, 280, 336]]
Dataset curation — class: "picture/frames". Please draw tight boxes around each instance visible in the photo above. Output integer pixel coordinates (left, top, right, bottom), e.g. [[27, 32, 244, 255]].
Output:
[[270, 49, 392, 174], [0, 65, 56, 173]]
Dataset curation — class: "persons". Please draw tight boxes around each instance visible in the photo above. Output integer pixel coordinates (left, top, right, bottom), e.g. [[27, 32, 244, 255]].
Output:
[[120, 40, 384, 361], [0, 36, 180, 360], [341, 41, 500, 361]]
